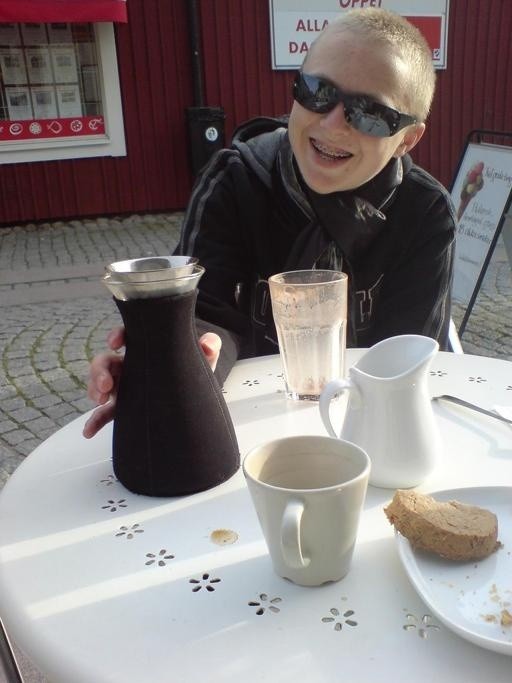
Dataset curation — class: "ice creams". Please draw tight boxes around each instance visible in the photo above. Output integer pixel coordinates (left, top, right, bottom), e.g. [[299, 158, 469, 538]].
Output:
[[457, 161, 485, 222]]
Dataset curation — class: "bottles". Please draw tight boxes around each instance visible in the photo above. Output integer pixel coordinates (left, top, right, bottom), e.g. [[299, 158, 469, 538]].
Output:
[[99, 255, 242, 500], [319, 334, 440, 490]]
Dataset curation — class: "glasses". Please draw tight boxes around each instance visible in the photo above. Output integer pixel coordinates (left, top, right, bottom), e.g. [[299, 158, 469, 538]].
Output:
[[290, 72, 418, 138]]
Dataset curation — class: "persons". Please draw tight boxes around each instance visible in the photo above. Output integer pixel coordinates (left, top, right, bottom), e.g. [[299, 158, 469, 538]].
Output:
[[82, 9, 458, 441]]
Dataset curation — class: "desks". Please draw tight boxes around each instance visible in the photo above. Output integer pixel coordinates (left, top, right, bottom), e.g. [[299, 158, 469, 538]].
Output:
[[1, 347, 512, 681]]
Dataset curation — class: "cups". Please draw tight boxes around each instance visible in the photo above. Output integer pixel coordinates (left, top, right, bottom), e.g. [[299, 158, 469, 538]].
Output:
[[240, 434, 372, 588], [267, 268, 350, 404]]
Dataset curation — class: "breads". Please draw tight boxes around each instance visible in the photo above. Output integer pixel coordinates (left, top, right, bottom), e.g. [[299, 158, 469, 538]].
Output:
[[384, 489, 497, 564]]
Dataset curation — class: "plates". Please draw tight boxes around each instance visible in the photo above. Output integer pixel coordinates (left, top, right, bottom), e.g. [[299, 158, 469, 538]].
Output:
[[395, 483, 512, 660]]
